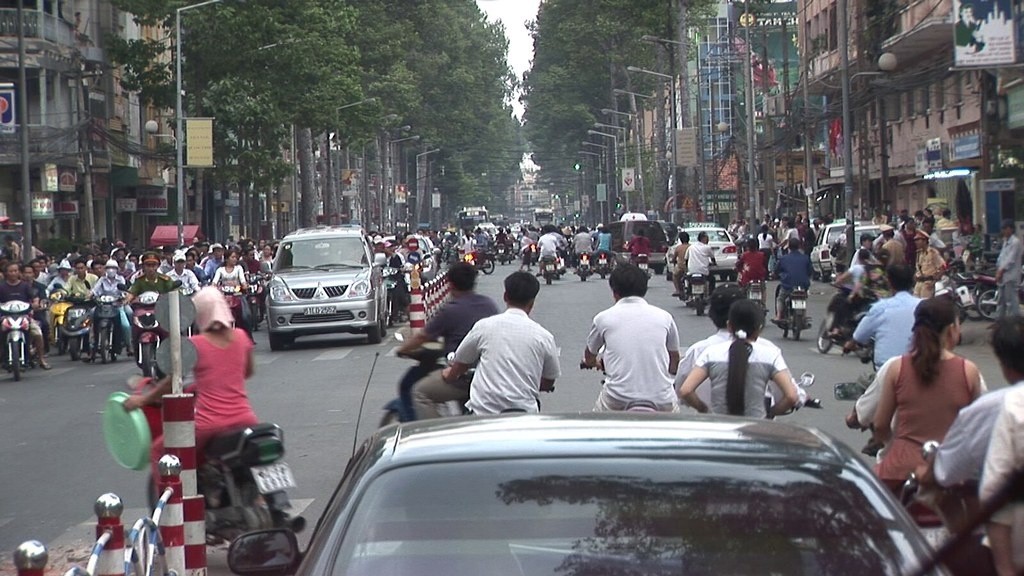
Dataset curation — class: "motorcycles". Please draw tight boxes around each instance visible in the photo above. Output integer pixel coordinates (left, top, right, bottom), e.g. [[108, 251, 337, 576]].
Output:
[[0, 204, 1024, 525], [126, 375, 305, 561]]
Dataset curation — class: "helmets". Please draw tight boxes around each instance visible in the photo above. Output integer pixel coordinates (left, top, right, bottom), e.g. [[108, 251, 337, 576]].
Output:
[[710, 282, 750, 315]]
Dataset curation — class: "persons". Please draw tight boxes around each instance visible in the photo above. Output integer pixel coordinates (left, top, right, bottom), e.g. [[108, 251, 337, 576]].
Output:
[[0, 217, 1024, 334], [382, 263, 806, 427], [843, 260, 989, 527], [122, 286, 260, 519], [396, 185, 407, 202], [914, 315, 1024, 576], [339, 170, 360, 194]]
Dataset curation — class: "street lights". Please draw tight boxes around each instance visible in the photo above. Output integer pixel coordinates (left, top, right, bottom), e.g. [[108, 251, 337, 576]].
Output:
[[335, 97, 440, 227], [576, 107, 631, 222], [612, 87, 657, 209], [627, 65, 677, 224], [641, 35, 709, 220]]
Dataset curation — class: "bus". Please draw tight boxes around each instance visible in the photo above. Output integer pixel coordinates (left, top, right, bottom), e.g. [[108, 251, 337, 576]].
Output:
[[531, 208, 556, 231], [458, 205, 490, 240]]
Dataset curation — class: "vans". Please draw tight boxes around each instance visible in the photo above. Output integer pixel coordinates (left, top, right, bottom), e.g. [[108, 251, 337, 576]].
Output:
[[260, 224, 390, 349]]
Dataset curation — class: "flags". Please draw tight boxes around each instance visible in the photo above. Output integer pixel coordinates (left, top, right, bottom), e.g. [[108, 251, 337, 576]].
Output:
[[735, 32, 779, 91], [776, 118, 792, 129], [827, 117, 841, 158]]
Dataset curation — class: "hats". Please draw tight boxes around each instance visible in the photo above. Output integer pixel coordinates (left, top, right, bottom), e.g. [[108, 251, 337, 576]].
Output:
[[879, 224, 895, 232], [173, 251, 187, 262], [373, 234, 385, 244], [860, 234, 877, 241], [104, 259, 119, 270], [56, 258, 73, 270], [208, 241, 227, 255], [141, 251, 162, 266], [1000, 218, 1014, 229], [913, 228, 930, 240]]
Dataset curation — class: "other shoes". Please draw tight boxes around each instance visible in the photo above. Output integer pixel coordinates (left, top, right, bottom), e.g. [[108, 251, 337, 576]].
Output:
[[39, 363, 52, 369], [83, 348, 96, 363], [246, 330, 257, 344], [125, 345, 135, 357]]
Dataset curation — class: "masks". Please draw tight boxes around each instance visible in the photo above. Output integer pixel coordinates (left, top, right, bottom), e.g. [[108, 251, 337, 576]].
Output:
[[105, 268, 118, 278]]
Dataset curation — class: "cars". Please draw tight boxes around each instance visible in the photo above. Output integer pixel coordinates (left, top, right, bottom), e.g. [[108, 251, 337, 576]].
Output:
[[226, 411, 955, 576], [810, 221, 906, 283], [664, 226, 739, 281]]
